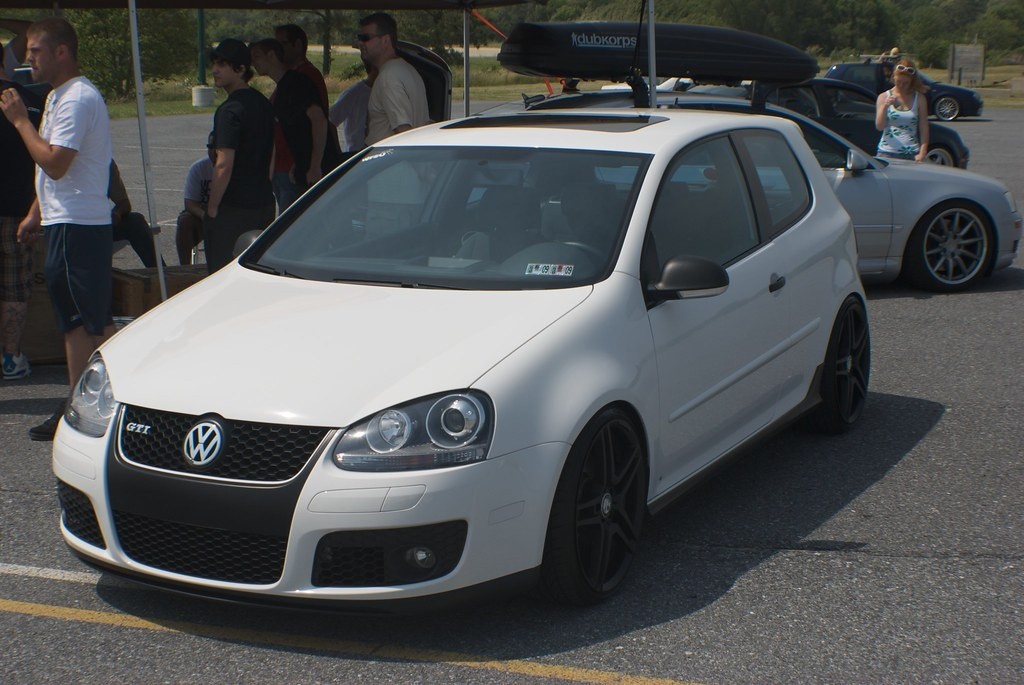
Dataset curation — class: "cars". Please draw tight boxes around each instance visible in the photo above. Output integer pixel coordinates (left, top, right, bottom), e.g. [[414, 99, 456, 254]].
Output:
[[821, 53, 985, 122], [512, 87, 1021, 291], [677, 76, 970, 175]]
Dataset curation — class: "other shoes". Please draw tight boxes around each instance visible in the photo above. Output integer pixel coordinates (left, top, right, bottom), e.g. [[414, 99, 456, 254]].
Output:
[[28, 407, 65, 441]]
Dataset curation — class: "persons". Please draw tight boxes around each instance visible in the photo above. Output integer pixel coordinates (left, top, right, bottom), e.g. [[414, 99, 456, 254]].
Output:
[[247, 24, 330, 216], [327, 12, 430, 165], [0, 18, 118, 441], [174, 130, 218, 266], [875, 60, 929, 162], [109, 157, 166, 268], [203, 39, 274, 276]]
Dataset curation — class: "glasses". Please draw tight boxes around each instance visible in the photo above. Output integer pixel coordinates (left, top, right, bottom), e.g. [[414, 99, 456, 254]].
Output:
[[357, 33, 382, 43], [895, 64, 916, 75], [206, 144, 216, 150]]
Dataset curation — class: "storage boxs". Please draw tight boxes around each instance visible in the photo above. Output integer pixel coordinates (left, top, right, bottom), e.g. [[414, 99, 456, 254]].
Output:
[[12, 224, 208, 364]]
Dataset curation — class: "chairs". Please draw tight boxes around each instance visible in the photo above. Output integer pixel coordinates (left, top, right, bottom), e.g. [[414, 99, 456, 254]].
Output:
[[435, 167, 751, 282]]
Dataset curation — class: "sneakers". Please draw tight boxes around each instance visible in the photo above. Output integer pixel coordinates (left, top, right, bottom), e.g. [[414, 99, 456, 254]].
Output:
[[2, 350, 32, 380]]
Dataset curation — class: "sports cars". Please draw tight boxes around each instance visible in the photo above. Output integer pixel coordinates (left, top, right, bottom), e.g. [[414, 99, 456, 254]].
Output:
[[50, 107, 873, 608]]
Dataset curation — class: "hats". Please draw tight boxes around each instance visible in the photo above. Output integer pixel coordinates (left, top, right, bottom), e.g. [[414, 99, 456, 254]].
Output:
[[210, 38, 253, 65]]
